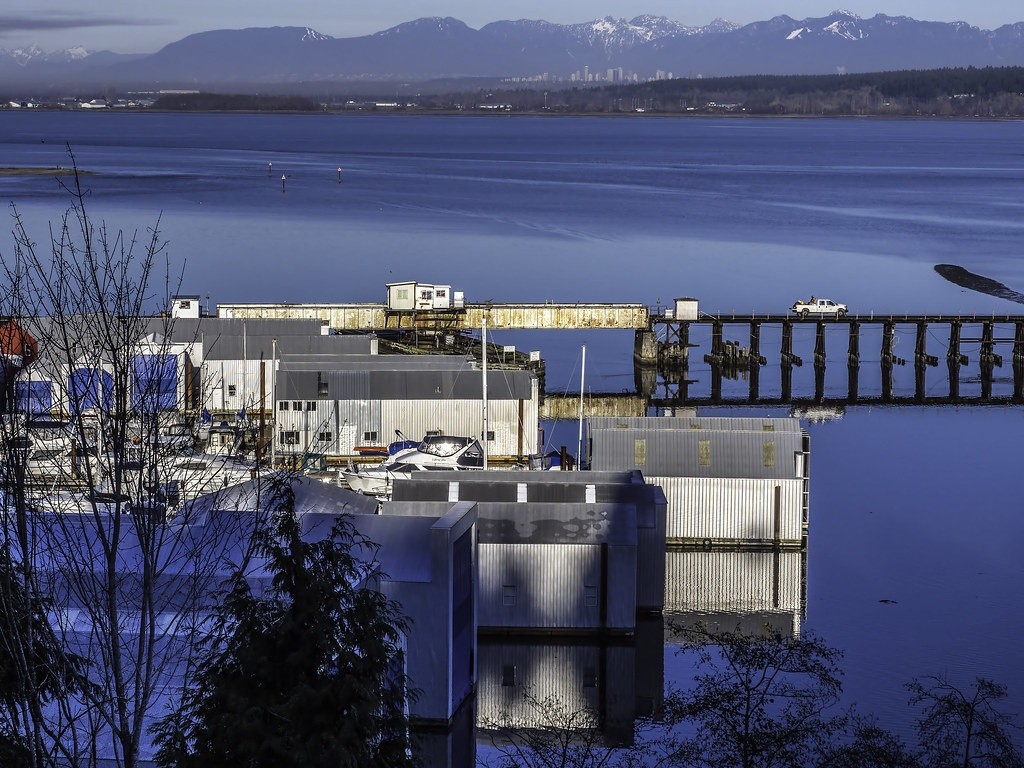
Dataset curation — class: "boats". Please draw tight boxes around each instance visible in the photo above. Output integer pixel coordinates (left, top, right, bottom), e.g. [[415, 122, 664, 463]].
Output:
[[1, 410, 522, 502]]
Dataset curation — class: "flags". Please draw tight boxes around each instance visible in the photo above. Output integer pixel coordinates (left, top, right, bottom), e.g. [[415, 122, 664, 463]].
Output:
[[237, 405, 246, 419], [203, 407, 210, 422]]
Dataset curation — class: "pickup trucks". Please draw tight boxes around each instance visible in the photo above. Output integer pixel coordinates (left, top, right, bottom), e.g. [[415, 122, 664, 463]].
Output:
[[790, 297, 849, 318]]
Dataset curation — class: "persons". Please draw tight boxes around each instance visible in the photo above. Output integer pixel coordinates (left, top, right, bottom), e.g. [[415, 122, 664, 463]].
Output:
[[808, 295, 816, 305]]
